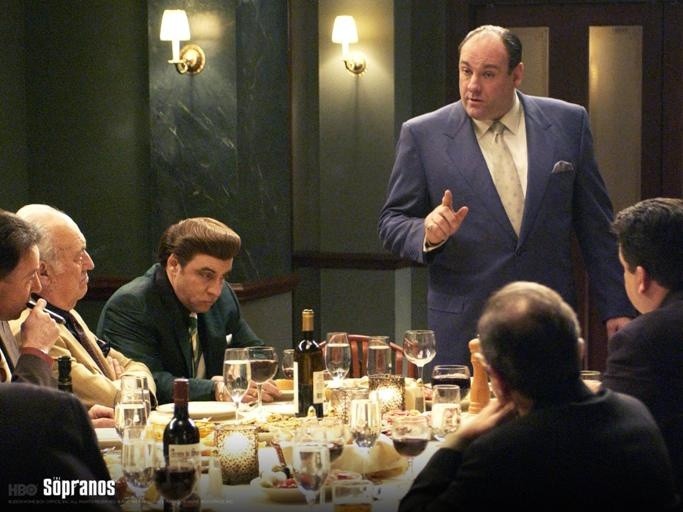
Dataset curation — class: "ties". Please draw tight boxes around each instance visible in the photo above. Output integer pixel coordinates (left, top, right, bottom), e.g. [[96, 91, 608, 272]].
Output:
[[488, 121, 525, 237], [0, 348, 14, 385], [187, 313, 207, 379], [65, 312, 107, 378]]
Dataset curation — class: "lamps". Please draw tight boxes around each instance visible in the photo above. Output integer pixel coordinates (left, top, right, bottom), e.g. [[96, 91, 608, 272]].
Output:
[[158, 9, 206, 77], [331, 15, 366, 74]]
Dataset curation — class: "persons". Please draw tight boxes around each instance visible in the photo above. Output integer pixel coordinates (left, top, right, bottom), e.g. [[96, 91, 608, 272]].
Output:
[[2, 201, 157, 411], [395, 277, 683, 509], [374, 22, 642, 388], [597, 194, 683, 511], [95, 214, 281, 401], [1, 208, 117, 430], [1, 353, 124, 512]]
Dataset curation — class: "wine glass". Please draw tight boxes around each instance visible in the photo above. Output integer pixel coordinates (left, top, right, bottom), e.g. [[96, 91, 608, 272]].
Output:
[[348, 388, 381, 501], [247, 345, 279, 415], [221, 348, 252, 419], [112, 373, 151, 438], [390, 416, 429, 480], [401, 328, 436, 386], [281, 349, 294, 379], [121, 426, 156, 511], [292, 427, 330, 511], [325, 331, 351, 388], [430, 364, 470, 405], [153, 455, 199, 509], [304, 420, 345, 511], [365, 334, 391, 378]]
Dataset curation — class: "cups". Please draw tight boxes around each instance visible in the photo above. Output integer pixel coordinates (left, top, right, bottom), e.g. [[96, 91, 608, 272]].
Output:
[[330, 479, 372, 512], [430, 385, 462, 443]]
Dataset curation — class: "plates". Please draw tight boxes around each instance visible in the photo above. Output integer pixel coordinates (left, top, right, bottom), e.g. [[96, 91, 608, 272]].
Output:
[[248, 470, 362, 503], [155, 400, 246, 420]]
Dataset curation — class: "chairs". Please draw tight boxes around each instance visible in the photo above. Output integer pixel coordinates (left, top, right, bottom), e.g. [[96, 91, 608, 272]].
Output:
[[319, 334, 417, 379]]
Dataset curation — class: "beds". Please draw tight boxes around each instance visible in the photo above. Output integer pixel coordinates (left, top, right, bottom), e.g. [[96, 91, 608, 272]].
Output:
[[96, 376, 494, 512]]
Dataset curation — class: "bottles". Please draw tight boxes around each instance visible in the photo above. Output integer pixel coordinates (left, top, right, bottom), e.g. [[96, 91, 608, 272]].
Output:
[[206, 447, 226, 499], [162, 377, 201, 512], [292, 308, 326, 418]]
